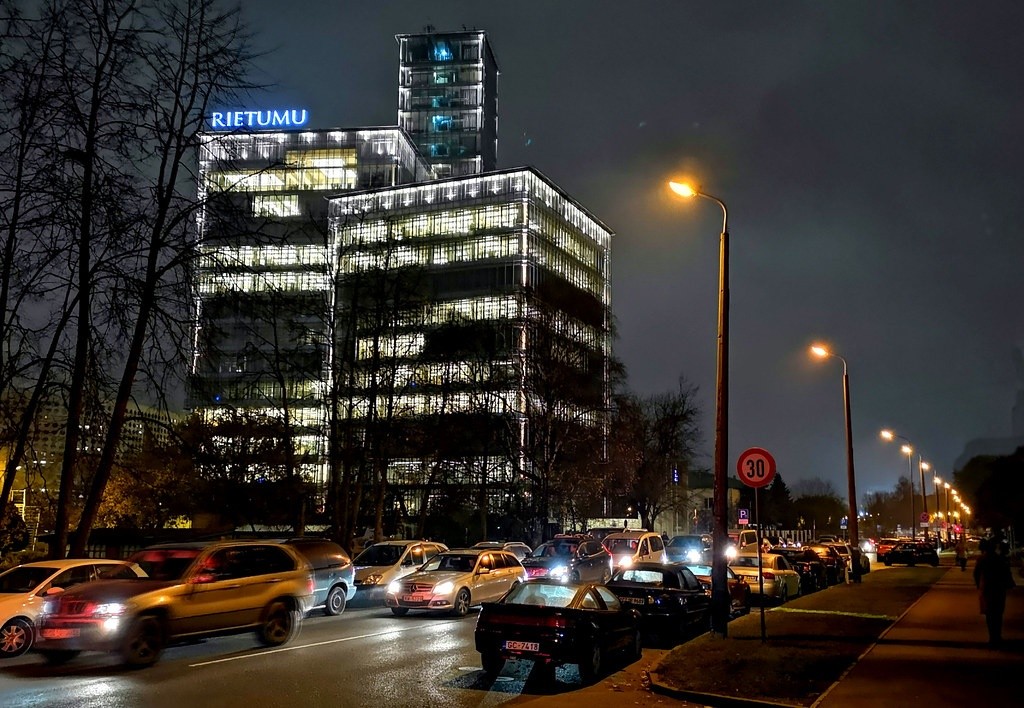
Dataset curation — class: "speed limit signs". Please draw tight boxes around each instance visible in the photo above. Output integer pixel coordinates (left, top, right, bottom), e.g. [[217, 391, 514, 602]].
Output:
[[737, 447, 777, 488]]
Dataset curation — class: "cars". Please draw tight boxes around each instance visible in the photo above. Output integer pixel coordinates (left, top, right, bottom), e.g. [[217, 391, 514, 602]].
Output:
[[859, 532, 982, 566], [473, 577, 643, 687], [352, 539, 450, 602], [0, 559, 148, 658], [474, 529, 877, 648], [386, 548, 528, 617]]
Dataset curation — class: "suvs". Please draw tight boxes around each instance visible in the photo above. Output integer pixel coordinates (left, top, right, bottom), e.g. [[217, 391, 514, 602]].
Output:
[[281, 539, 358, 619], [37, 540, 319, 670]]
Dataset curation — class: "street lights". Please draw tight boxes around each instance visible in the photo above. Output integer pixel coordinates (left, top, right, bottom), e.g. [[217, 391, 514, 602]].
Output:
[[812, 347, 861, 583], [921, 461, 971, 552], [902, 445, 928, 541], [669, 178, 728, 634], [881, 430, 915, 542]]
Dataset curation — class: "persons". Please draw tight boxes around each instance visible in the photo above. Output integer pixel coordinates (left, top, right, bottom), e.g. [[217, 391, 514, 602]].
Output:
[[972, 543, 1016, 642], [956, 536, 968, 571]]
